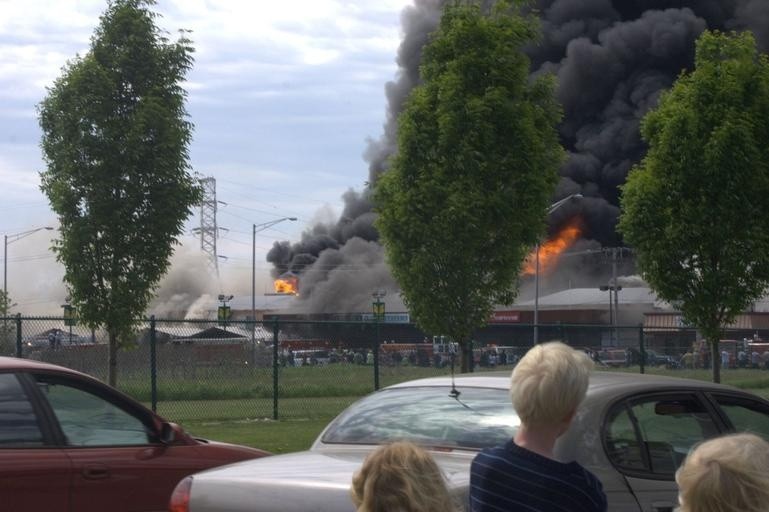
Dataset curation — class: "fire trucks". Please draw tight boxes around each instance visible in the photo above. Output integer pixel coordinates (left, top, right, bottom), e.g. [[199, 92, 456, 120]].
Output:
[[697, 339, 768, 368]]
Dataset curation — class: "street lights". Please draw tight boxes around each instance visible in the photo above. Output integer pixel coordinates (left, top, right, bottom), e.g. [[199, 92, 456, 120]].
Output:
[[2, 226, 58, 348], [532, 194, 581, 342], [253, 217, 297, 361]]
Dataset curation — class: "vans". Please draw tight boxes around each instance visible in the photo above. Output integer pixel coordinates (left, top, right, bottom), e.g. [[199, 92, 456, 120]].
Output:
[[598, 349, 627, 366]]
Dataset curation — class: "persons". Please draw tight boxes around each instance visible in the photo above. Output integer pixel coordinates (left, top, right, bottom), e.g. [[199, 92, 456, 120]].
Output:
[[351, 440, 463, 511], [678, 331, 769, 369], [469, 340, 609, 511], [279, 346, 440, 369], [480, 351, 519, 367], [674, 432, 768, 511]]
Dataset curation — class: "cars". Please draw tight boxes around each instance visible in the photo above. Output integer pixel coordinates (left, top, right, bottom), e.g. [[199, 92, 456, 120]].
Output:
[[165, 366, 766, 512], [1, 356, 270, 512]]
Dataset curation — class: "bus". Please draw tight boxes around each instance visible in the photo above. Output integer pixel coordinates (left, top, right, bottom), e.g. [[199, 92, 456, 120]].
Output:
[[490, 346, 521, 365], [277, 349, 332, 366]]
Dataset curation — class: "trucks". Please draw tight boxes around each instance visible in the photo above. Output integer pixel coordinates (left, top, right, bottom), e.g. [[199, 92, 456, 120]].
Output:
[[381, 334, 459, 361]]
